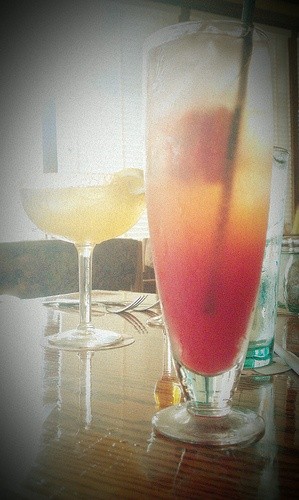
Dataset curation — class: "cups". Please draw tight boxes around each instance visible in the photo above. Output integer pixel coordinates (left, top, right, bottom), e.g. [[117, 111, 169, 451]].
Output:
[[239, 143, 293, 378], [275, 234, 299, 316]]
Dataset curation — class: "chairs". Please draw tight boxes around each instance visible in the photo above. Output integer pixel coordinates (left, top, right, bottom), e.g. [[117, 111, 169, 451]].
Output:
[[87, 237, 156, 294], [0, 239, 82, 299]]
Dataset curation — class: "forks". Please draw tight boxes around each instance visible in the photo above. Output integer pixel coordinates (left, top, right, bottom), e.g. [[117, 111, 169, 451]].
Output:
[[47, 305, 160, 335], [42, 294, 161, 314]]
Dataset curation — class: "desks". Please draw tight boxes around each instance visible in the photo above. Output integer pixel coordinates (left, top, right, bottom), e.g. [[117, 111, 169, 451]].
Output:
[[1, 290, 299, 500]]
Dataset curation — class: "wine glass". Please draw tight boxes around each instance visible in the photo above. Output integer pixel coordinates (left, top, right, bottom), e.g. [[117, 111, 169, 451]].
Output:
[[137, 16, 270, 446], [30, 336, 149, 499], [143, 321, 186, 411], [14, 164, 145, 352]]
